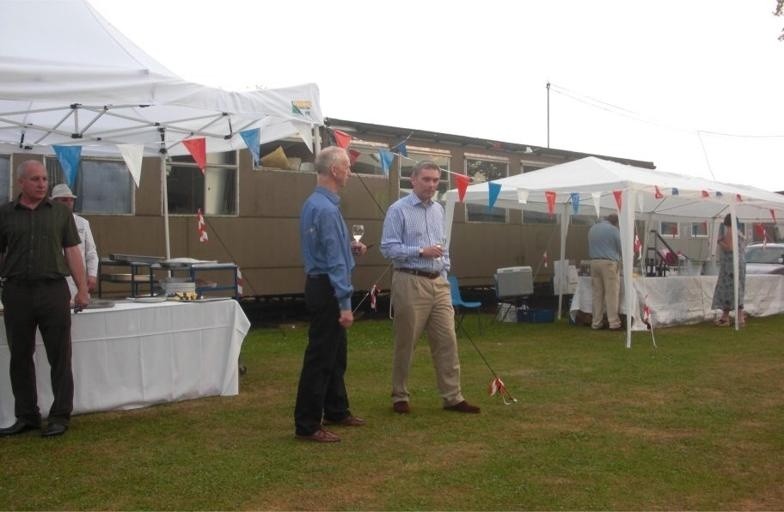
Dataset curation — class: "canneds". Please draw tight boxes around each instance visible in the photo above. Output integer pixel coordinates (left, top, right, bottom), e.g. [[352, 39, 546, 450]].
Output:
[[433, 244, 442, 261]]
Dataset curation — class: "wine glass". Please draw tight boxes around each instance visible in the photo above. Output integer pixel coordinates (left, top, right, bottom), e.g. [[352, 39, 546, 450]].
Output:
[[350, 224, 364, 242]]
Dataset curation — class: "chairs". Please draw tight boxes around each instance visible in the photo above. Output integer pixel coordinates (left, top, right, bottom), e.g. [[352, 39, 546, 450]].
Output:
[[446, 276, 481, 333]]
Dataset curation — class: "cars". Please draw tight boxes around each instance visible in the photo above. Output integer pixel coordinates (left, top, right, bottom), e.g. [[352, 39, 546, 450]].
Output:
[[746, 242, 784, 275]]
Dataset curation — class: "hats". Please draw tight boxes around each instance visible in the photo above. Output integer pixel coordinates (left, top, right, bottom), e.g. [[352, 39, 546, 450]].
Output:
[[48, 183, 79, 201]]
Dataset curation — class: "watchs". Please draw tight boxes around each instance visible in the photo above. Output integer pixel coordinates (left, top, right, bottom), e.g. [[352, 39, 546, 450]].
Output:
[[419, 247, 424, 257]]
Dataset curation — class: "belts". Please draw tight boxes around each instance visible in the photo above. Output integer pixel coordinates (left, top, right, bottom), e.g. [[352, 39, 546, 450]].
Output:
[[591, 257, 611, 260], [394, 267, 440, 280]]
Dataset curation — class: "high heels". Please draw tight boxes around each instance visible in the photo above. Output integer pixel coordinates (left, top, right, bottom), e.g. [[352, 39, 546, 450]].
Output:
[[713, 318, 730, 327], [738, 319, 748, 328]]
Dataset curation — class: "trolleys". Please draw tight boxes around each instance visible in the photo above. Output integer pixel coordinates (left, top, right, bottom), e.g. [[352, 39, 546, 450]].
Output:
[[149, 261, 252, 375]]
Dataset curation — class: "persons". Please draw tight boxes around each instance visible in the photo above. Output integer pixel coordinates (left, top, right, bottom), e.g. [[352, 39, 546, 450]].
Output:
[[711, 214, 749, 327], [379, 159, 481, 413], [295, 146, 366, 442], [587, 213, 623, 331], [1, 159, 91, 436], [48, 184, 99, 304]]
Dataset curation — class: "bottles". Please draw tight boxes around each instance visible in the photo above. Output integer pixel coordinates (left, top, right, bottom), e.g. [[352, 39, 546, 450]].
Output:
[[645, 257, 655, 276]]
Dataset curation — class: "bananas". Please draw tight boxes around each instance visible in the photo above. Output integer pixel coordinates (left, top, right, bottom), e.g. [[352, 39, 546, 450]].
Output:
[[173, 290, 198, 300]]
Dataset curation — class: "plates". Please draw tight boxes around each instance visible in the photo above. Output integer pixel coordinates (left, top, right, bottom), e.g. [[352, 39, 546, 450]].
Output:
[[135, 297, 167, 304]]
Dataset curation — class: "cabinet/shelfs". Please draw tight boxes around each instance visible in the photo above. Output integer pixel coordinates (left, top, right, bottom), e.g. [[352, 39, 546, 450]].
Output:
[[149, 264, 237, 300], [98, 259, 156, 300]]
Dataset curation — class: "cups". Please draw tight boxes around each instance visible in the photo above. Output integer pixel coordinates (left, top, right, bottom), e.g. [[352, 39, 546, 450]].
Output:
[[432, 239, 447, 259]]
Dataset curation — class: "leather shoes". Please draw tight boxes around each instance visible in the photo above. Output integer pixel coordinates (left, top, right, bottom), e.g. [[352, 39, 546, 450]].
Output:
[[295, 425, 341, 443], [322, 413, 368, 426], [591, 321, 626, 330], [40, 421, 68, 436], [443, 400, 481, 413], [0, 415, 41, 435], [393, 399, 410, 412]]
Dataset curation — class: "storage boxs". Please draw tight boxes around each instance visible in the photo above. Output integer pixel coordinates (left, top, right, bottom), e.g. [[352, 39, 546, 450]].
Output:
[[496, 266, 533, 298]]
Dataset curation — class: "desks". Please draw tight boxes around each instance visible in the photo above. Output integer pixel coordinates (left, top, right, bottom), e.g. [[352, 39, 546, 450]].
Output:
[[494, 299, 531, 323], [568, 272, 784, 332], [2, 291, 250, 429]]
